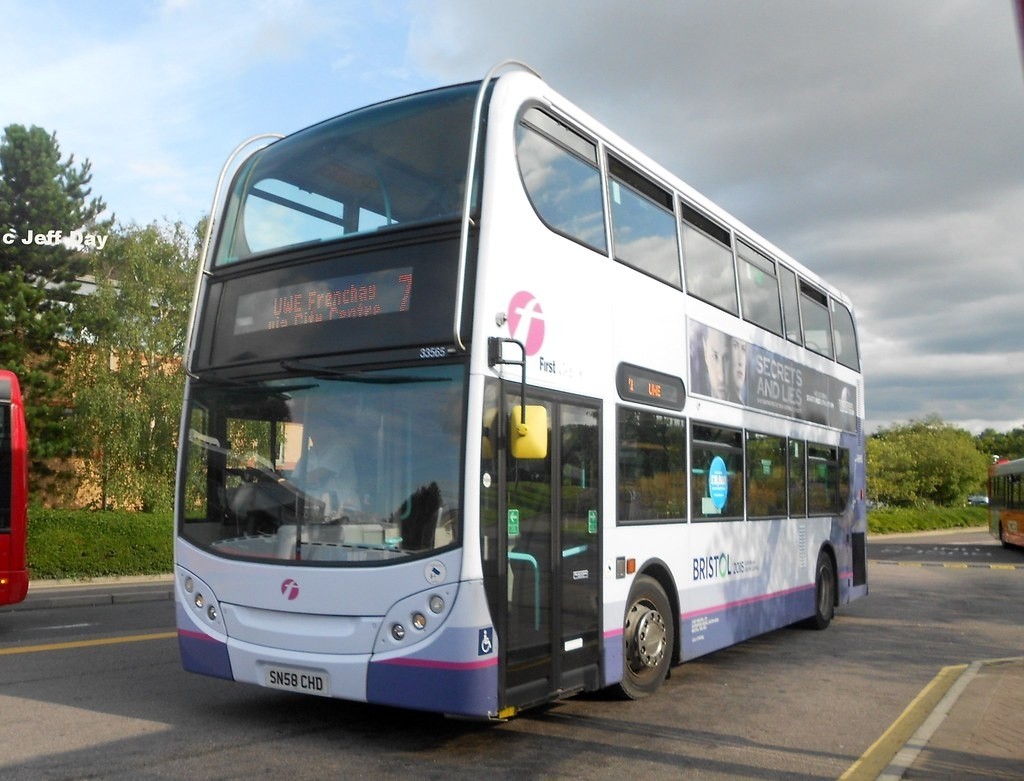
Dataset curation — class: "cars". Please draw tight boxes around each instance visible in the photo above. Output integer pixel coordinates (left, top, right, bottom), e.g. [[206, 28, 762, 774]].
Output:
[[967, 495, 989, 505]]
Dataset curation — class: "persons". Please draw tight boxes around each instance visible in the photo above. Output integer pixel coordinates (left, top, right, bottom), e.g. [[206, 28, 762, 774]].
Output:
[[282, 415, 362, 515], [701, 325, 747, 407]]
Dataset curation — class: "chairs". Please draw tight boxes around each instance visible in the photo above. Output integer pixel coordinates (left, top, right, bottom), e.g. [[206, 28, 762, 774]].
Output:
[[348, 431, 379, 515], [616, 489, 846, 521]]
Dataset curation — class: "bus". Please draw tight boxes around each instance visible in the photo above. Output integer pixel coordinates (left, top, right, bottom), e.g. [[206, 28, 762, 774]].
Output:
[[174, 59, 868, 722], [0, 370, 29, 606], [986, 455, 1024, 548]]
